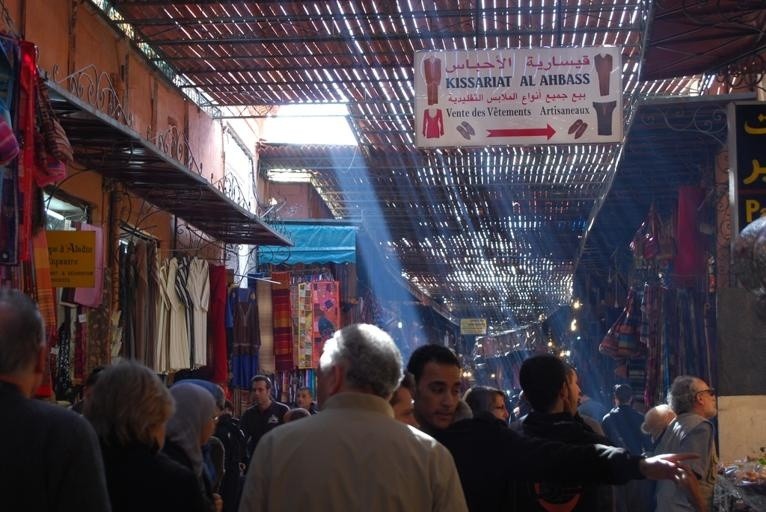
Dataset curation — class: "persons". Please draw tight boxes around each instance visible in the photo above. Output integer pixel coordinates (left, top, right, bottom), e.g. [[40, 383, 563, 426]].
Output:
[[69, 322, 723, 511], [0, 284, 112, 511]]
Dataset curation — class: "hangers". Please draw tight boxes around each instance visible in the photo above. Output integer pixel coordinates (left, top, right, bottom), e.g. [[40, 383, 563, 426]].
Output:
[[119, 225, 153, 244], [163, 249, 205, 266]]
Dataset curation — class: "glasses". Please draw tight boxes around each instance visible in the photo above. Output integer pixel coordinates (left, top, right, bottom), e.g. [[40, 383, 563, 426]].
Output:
[[695, 388, 715, 398]]
